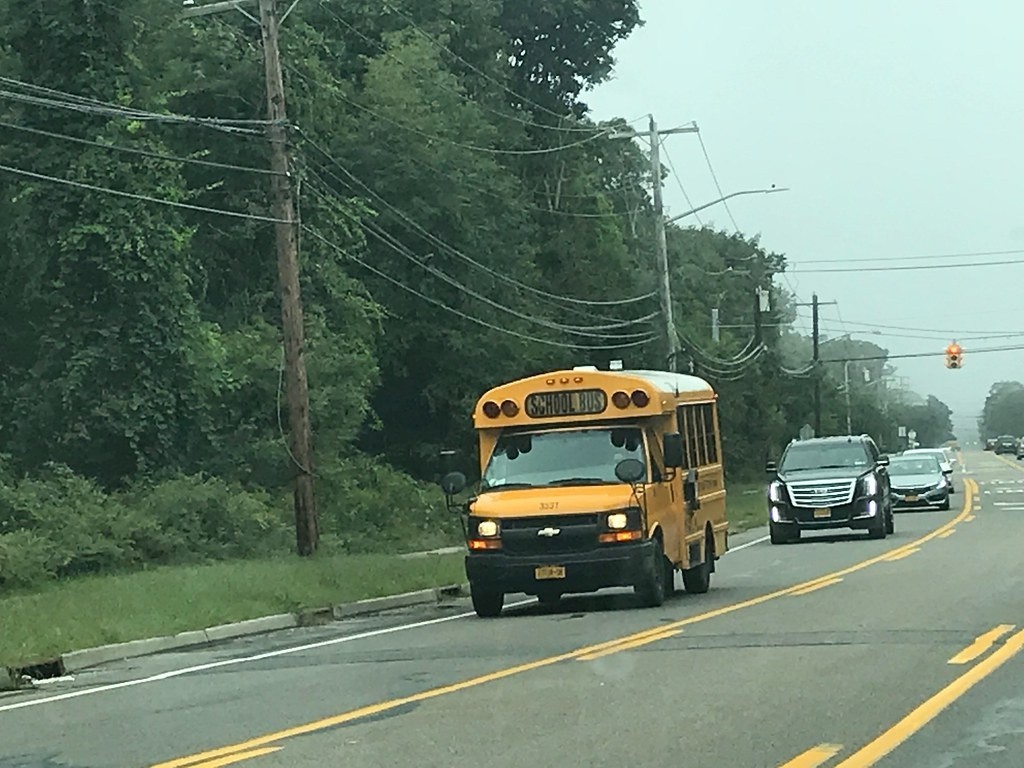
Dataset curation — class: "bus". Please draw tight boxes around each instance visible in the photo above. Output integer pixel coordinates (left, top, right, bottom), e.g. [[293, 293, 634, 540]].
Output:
[[444, 359, 727, 621]]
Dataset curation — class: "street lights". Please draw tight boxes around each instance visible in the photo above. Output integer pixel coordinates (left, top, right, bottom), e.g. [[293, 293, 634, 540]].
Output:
[[650, 184, 791, 364], [809, 329, 886, 430]]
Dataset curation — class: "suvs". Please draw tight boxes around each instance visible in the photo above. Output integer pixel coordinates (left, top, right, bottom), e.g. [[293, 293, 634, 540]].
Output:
[[764, 433, 895, 545]]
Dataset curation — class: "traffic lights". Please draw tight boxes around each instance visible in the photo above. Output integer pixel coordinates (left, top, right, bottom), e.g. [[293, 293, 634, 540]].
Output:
[[948, 344, 961, 371]]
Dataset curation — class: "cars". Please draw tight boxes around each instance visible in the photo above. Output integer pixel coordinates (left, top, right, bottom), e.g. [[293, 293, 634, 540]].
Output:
[[884, 455, 952, 512], [903, 449, 957, 494], [986, 435, 1024, 461]]
